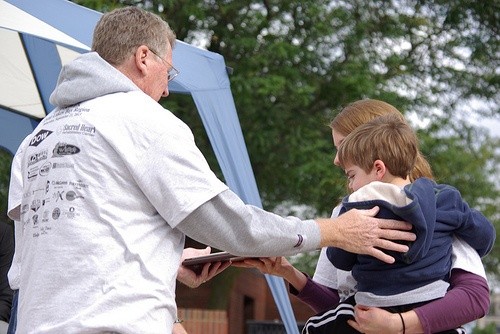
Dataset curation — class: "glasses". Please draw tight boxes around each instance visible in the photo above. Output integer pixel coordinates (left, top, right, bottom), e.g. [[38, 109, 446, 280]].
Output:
[[133, 48, 180, 82]]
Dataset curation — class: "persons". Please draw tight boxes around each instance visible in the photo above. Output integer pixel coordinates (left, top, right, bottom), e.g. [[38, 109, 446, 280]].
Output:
[[232, 100, 490, 334], [300, 115, 497, 334], [7, 8, 418, 334]]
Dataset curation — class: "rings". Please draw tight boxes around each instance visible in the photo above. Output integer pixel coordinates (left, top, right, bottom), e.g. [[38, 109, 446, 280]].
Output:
[[203, 280, 206, 283]]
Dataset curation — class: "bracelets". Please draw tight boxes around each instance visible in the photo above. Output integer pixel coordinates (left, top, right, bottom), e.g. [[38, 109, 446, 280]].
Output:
[[398, 313, 406, 334], [174, 319, 183, 324]]
